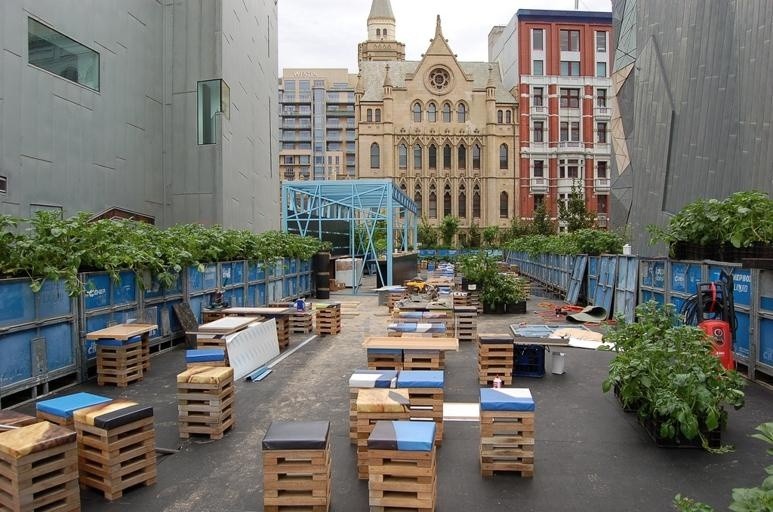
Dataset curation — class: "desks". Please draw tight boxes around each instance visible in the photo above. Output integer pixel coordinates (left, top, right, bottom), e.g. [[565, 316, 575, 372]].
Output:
[[260, 417, 332, 512], [0, 390, 158, 512], [349, 283, 535, 512], [84, 322, 158, 385], [176, 298, 344, 445]]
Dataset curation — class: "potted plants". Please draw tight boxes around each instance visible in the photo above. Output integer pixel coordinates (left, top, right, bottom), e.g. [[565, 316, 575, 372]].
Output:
[[624, 326, 746, 448], [644, 190, 773, 265], [599, 299, 681, 412], [458, 252, 526, 314]]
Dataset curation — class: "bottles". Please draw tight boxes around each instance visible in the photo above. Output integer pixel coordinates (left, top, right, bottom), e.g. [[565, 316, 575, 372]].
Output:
[[295, 298, 306, 312]]
